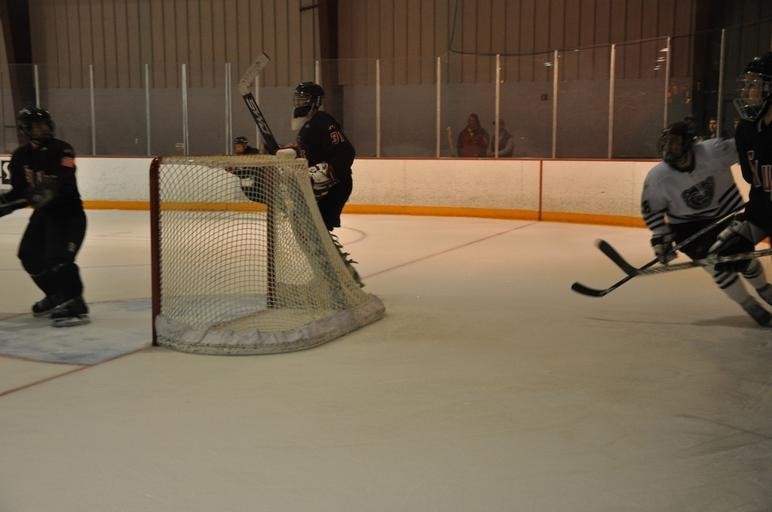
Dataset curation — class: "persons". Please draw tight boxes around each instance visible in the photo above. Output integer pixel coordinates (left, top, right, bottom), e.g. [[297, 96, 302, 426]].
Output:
[[0, 107, 90, 319], [707, 56, 772, 272], [641, 120, 772, 327], [490, 119, 513, 157], [458, 113, 490, 157], [232, 136, 258, 155], [708, 117, 732, 140], [278, 81, 362, 284]]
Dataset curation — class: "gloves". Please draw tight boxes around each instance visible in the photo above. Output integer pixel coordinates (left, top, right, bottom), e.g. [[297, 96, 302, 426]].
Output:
[[651, 233, 678, 266], [709, 218, 766, 276], [27, 178, 61, 210], [306, 161, 338, 197]]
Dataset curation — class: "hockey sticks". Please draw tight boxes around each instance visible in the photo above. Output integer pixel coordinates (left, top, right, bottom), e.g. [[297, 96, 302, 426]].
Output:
[[238, 52, 282, 153], [571, 204, 746, 297], [596, 237, 772, 276]]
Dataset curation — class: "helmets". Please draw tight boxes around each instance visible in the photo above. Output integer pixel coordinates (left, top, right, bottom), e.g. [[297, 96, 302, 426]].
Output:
[[14, 106, 54, 144], [293, 82, 326, 115], [658, 125, 695, 162], [734, 55, 770, 121], [231, 136, 249, 145]]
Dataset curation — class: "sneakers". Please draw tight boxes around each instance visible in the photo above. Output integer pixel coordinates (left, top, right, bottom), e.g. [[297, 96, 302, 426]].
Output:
[[748, 304, 772, 328], [31, 291, 59, 317], [49, 295, 91, 329], [344, 261, 365, 288]]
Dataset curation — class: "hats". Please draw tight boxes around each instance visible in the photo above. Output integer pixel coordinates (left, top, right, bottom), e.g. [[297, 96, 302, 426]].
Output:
[[494, 119, 506, 126]]
